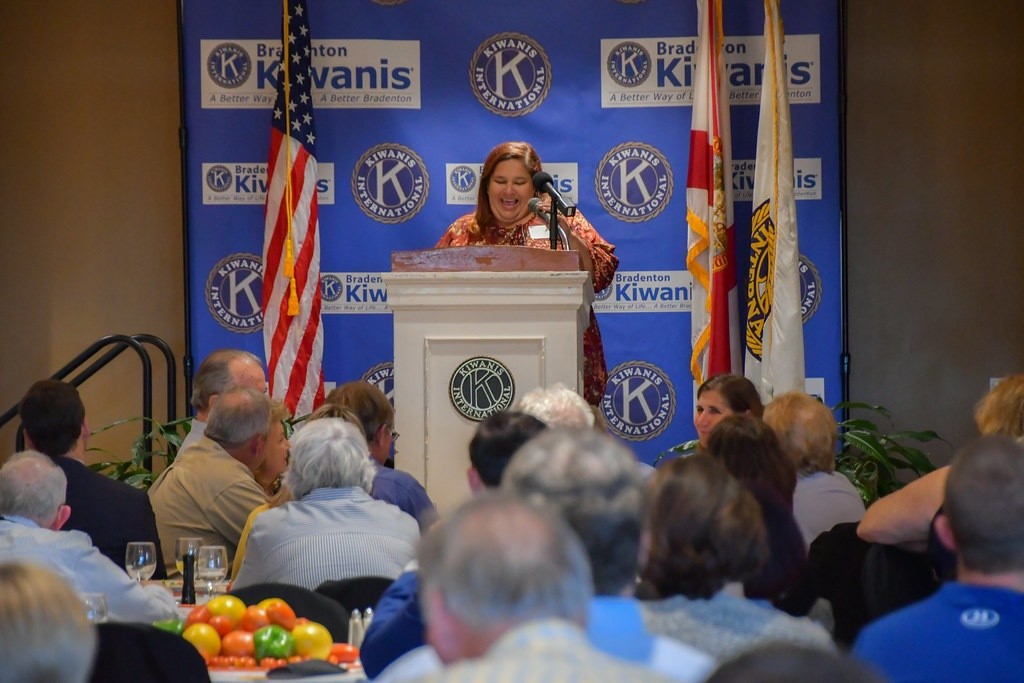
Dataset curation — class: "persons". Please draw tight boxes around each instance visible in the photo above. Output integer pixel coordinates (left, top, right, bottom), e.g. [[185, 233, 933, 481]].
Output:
[[230, 383, 437, 591], [19, 378, 168, 580], [418, 489, 660, 682], [1, 560, 98, 683], [850, 433, 1024, 683], [0, 450, 178, 624], [176, 350, 269, 456], [434, 142, 620, 407], [254, 400, 292, 487], [857, 374, 1024, 550], [148, 391, 273, 579], [359, 374, 874, 683]]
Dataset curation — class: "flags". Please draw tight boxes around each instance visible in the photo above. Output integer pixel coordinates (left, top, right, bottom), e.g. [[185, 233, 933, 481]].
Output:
[[687, 0, 743, 416], [263, 0, 325, 418], [745, 0, 804, 404]]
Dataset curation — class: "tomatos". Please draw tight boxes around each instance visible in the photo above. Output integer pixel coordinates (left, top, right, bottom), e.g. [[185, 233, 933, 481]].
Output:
[[181, 596, 359, 663]]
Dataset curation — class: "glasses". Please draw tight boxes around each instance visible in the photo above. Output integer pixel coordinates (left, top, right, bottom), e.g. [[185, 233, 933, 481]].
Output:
[[377, 424, 399, 442]]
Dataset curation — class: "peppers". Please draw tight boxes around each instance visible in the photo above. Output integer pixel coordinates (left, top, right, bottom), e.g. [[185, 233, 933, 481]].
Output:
[[154, 618, 185, 636], [253, 624, 295, 663]]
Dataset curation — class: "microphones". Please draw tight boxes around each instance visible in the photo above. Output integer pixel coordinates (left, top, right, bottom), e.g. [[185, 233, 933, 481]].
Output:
[[526, 198, 570, 251], [533, 171, 576, 217]]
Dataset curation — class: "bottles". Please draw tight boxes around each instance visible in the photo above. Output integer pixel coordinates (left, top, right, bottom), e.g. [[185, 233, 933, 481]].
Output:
[[363, 607, 374, 635], [348, 609, 363, 648]]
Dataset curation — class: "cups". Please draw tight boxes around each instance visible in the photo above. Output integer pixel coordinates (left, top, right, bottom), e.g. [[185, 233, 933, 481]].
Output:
[[125, 541, 156, 581]]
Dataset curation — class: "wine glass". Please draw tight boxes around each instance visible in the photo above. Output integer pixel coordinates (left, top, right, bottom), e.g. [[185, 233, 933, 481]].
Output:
[[176, 537, 205, 592], [198, 546, 228, 600]]
